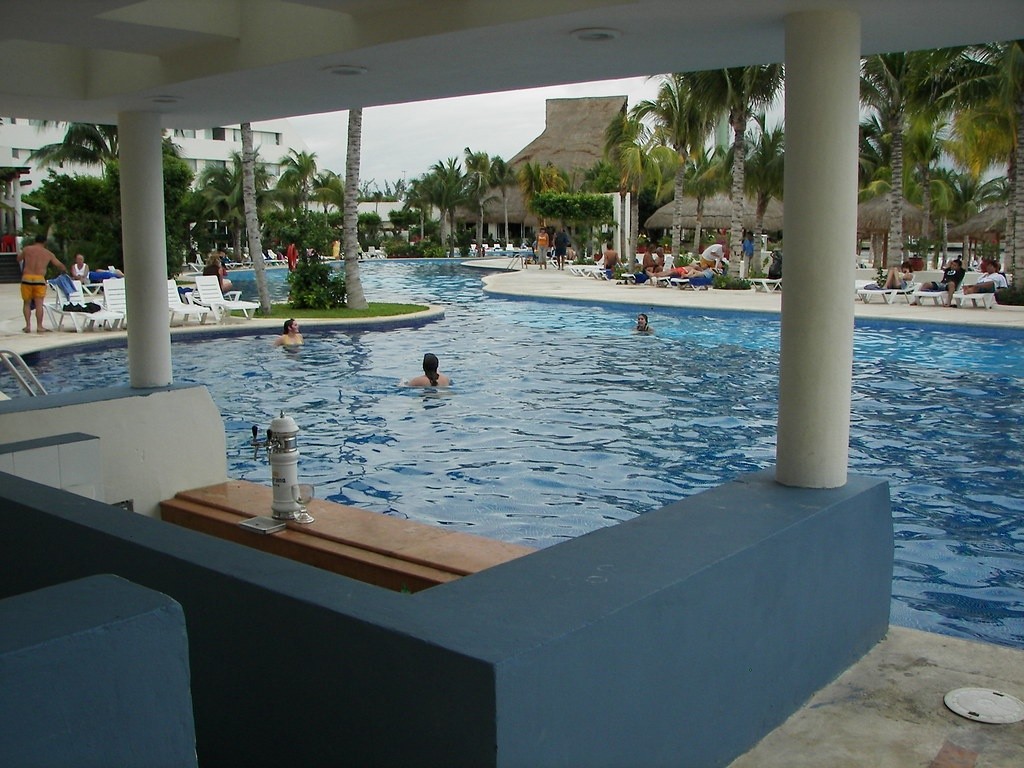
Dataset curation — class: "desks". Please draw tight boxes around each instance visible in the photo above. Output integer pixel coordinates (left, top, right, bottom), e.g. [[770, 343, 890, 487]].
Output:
[[158, 478, 539, 595]]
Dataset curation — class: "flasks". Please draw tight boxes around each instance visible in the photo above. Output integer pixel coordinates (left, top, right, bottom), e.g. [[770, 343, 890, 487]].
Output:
[[269, 410, 300, 519]]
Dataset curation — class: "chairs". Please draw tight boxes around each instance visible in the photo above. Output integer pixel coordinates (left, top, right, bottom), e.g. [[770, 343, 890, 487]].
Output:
[[185, 275, 261, 325], [43, 280, 124, 334], [913, 273, 966, 307], [747, 278, 782, 294], [953, 274, 1011, 310], [858, 273, 921, 304], [104, 279, 127, 329], [547, 255, 611, 281], [188, 249, 288, 272], [365, 246, 387, 259], [470, 244, 491, 252], [168, 280, 210, 327], [505, 244, 514, 251], [622, 256, 729, 292], [493, 244, 502, 252]]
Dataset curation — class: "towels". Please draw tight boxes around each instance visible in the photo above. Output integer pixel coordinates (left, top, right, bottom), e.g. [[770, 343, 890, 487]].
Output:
[[48, 272, 78, 301]]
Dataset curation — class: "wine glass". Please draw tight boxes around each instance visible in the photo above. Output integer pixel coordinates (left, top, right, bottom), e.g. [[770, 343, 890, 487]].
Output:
[[291, 485, 315, 524]]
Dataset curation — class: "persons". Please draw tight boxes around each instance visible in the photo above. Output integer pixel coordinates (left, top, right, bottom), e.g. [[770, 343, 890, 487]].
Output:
[[187, 237, 363, 271], [203, 254, 232, 294], [408, 352, 450, 385], [871, 262, 916, 290], [962, 260, 1008, 295], [70, 253, 89, 285], [521, 225, 783, 288], [16, 233, 67, 333], [273, 318, 304, 345], [908, 259, 966, 307], [957, 255, 1002, 273], [632, 313, 655, 333]]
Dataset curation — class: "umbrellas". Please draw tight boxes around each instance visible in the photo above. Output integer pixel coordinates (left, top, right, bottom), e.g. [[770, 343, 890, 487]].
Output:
[[946, 201, 1010, 262], [856, 190, 935, 270], [643, 186, 784, 234]]
